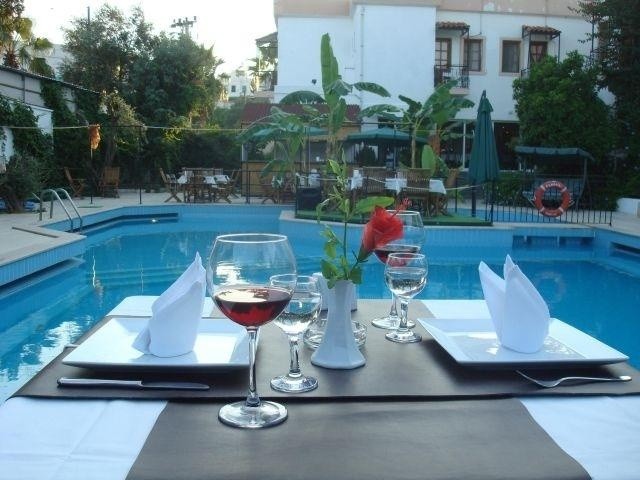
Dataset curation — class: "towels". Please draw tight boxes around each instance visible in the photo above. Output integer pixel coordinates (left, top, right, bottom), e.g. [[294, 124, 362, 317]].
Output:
[[477, 251, 553, 353], [134, 252, 210, 357]]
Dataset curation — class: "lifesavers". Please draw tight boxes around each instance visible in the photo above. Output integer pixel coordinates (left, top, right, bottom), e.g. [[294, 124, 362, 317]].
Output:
[[533, 180, 570, 217]]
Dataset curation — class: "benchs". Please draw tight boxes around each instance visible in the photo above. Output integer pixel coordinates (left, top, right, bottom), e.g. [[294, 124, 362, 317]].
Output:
[[521, 176, 593, 211]]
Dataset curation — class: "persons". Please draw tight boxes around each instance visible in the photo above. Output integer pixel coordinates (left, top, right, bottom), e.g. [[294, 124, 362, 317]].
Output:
[[385, 148, 394, 171]]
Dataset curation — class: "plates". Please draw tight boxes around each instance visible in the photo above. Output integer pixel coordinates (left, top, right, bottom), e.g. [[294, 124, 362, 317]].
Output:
[[60, 315, 262, 377], [415, 313, 631, 379]]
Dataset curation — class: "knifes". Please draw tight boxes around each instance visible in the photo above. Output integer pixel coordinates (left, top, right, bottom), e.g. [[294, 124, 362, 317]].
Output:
[[54, 374, 211, 392]]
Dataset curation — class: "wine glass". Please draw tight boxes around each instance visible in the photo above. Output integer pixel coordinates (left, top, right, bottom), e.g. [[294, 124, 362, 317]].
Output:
[[372, 208, 426, 333], [382, 251, 429, 345], [264, 272, 323, 396], [202, 232, 299, 432]]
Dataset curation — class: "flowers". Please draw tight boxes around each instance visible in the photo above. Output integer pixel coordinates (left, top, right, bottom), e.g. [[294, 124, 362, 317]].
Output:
[[312, 147, 414, 289]]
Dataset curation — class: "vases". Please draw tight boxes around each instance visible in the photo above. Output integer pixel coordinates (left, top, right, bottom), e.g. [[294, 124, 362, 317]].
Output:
[[310, 278, 368, 370]]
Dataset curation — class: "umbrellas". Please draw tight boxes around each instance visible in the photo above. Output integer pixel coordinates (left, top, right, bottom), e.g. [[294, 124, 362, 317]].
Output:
[[348, 126, 429, 171], [251, 124, 329, 176], [468, 90, 501, 221]]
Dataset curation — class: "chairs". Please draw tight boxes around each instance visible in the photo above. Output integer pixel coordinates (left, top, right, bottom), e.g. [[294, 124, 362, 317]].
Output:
[[157, 165, 241, 204], [254, 174, 295, 205], [317, 167, 461, 218], [63, 165, 124, 198]]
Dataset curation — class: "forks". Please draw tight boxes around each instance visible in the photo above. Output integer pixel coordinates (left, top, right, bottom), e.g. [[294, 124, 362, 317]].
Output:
[[513, 366, 632, 390]]
